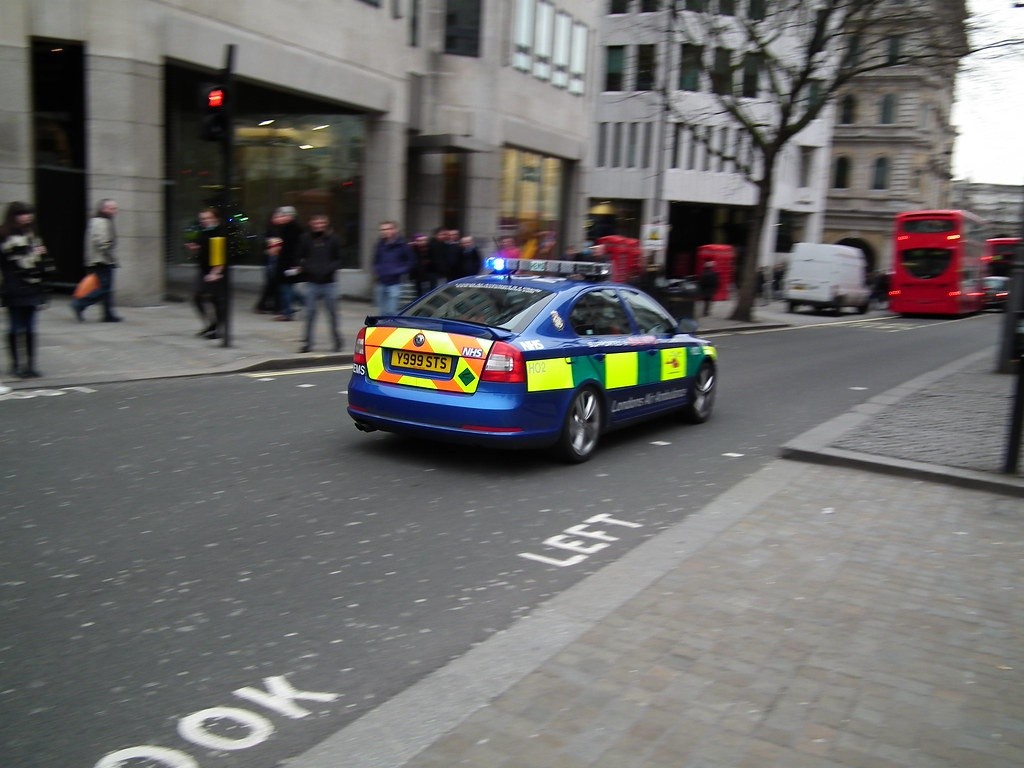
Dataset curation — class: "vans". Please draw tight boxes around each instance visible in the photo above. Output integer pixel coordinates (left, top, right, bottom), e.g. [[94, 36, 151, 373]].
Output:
[[782, 243, 873, 316]]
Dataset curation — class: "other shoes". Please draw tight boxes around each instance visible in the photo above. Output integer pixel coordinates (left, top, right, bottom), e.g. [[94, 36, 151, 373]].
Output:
[[101, 314, 121, 322], [196, 322, 228, 339], [72, 303, 86, 322], [10, 365, 43, 377]]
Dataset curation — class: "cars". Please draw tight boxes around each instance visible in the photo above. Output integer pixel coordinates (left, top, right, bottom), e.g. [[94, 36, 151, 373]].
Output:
[[983, 237, 1024, 270], [982, 276, 1009, 309], [346, 257, 719, 467]]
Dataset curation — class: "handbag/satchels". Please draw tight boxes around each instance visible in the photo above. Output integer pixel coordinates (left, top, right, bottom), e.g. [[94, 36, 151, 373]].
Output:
[[73, 274, 101, 298]]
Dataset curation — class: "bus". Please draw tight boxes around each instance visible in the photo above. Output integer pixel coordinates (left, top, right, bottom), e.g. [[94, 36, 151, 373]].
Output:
[[889, 209, 986, 317]]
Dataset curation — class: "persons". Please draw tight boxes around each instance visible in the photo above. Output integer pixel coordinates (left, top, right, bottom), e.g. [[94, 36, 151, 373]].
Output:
[[407, 227, 481, 296], [370, 221, 409, 315], [294, 213, 345, 353], [70, 198, 123, 323], [0, 200, 56, 378], [255, 205, 302, 321], [495, 235, 521, 258], [194, 203, 229, 338], [576, 244, 608, 282], [698, 257, 719, 316]]
